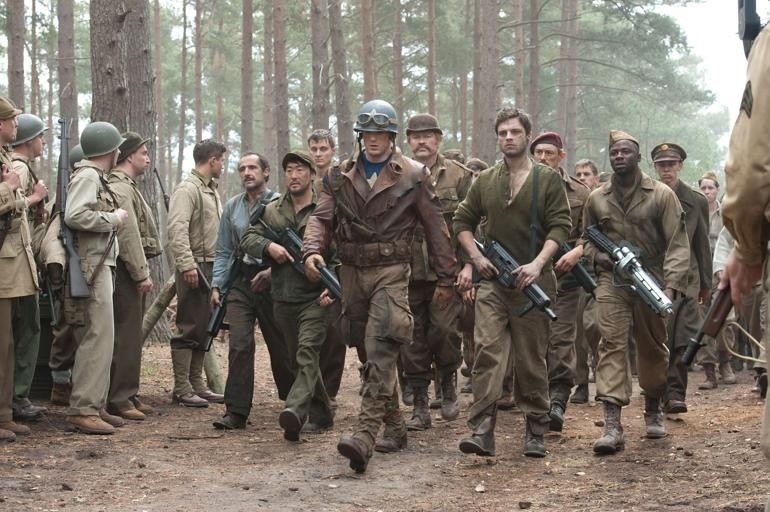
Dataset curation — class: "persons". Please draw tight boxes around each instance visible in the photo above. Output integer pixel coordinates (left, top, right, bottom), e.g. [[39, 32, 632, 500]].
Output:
[[717, 21, 770, 461], [459, 159, 490, 394], [442, 149, 463, 163], [698, 172, 736, 390], [403, 114, 474, 431], [107, 131, 163, 420], [569, 159, 600, 404], [64, 121, 128, 435], [0, 96, 36, 443], [307, 129, 368, 409], [211, 153, 298, 430], [582, 130, 688, 455], [651, 143, 713, 415], [49, 143, 91, 406], [452, 107, 570, 458], [239, 153, 342, 442], [11, 114, 48, 420], [166, 139, 227, 407], [598, 172, 613, 183], [300, 100, 458, 474], [529, 131, 592, 431]]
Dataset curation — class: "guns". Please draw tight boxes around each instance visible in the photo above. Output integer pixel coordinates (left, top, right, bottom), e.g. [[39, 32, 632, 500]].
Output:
[[58, 113, 90, 298], [585, 224, 676, 318], [42, 259, 58, 329], [153, 167, 212, 295], [250, 203, 344, 298], [553, 242, 598, 302], [681, 282, 733, 366], [471, 239, 557, 321], [202, 258, 241, 351]]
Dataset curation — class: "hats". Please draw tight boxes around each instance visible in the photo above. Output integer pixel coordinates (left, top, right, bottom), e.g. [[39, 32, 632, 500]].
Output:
[[0, 95, 23, 121], [80, 120, 126, 158], [529, 131, 564, 154], [465, 157, 489, 173], [115, 132, 150, 164], [650, 143, 688, 164], [8, 114, 51, 147], [697, 171, 719, 187], [606, 129, 641, 148], [281, 150, 317, 173], [70, 144, 87, 171], [405, 114, 443, 138], [350, 100, 401, 136]]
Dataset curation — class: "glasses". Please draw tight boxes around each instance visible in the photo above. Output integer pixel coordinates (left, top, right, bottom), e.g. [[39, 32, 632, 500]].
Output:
[[354, 113, 400, 129]]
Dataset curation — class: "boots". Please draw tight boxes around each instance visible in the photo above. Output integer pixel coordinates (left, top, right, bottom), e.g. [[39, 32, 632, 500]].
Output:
[[439, 372, 460, 420], [430, 368, 443, 407], [550, 397, 565, 432], [718, 360, 736, 386], [697, 365, 718, 390], [591, 402, 626, 453], [171, 348, 209, 407], [405, 386, 432, 431], [642, 396, 668, 439], [189, 350, 226, 403], [569, 384, 589, 404], [397, 368, 415, 406], [523, 418, 547, 457], [457, 414, 497, 457]]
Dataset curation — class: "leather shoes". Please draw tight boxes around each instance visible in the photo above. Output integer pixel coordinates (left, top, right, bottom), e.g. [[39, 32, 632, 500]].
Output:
[[277, 407, 304, 442], [65, 414, 116, 436], [13, 407, 43, 422], [99, 408, 123, 427], [135, 403, 154, 415], [0, 428, 17, 442], [336, 437, 372, 474], [50, 384, 70, 406], [1, 422, 30, 437], [373, 435, 409, 452], [111, 409, 148, 420], [212, 414, 248, 431], [460, 378, 475, 393], [660, 400, 687, 415], [24, 404, 49, 417]]
